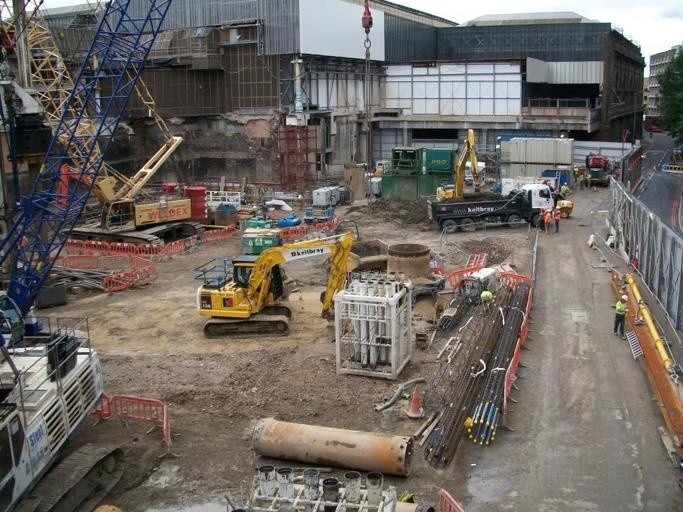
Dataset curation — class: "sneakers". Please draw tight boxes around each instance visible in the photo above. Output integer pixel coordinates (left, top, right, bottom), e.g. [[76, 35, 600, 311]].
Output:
[[614, 332, 625, 340]]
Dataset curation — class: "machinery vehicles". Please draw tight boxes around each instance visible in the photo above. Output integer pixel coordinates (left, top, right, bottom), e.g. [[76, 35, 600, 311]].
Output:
[[382, 125, 480, 200], [194, 233, 355, 337]]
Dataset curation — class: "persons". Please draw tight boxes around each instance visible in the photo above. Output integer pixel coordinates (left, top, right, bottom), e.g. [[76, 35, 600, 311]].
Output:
[[481, 290, 493, 311], [573, 166, 592, 190], [613, 295, 629, 340], [493, 182, 502, 193], [536, 179, 570, 235]]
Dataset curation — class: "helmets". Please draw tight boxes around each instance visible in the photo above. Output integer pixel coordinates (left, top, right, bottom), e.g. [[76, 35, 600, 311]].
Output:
[[547, 209, 551, 212], [556, 206, 561, 209], [621, 295, 628, 301]]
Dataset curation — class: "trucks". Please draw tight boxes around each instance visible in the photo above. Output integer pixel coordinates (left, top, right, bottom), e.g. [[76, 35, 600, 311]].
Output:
[[427, 183, 556, 234]]
